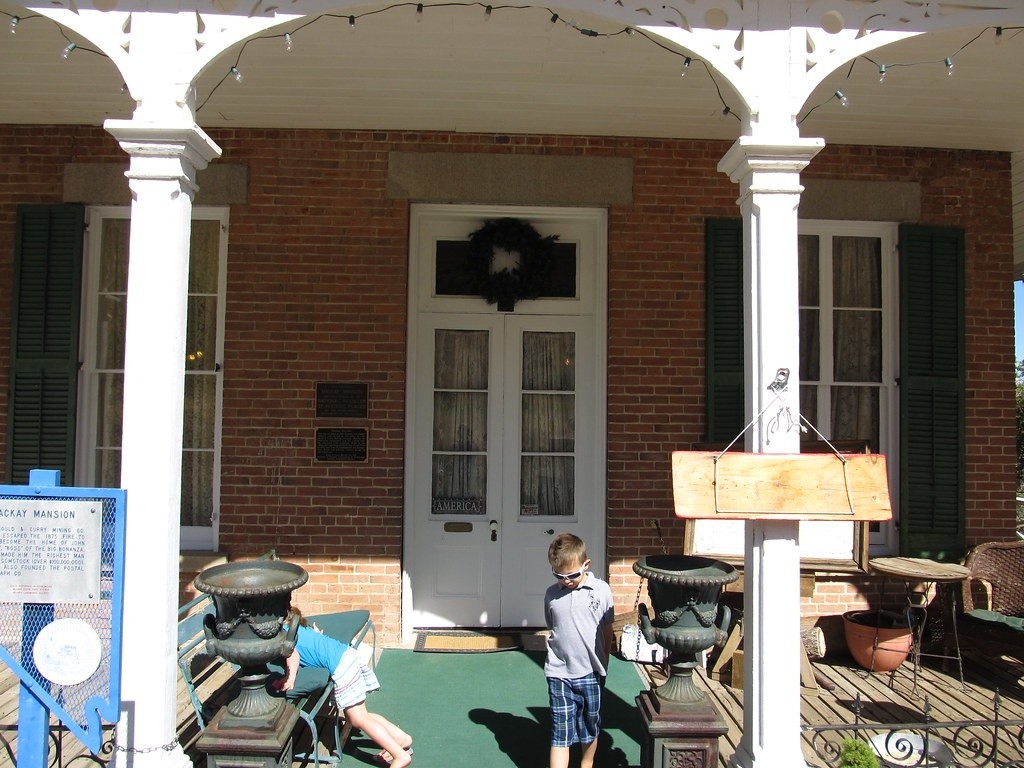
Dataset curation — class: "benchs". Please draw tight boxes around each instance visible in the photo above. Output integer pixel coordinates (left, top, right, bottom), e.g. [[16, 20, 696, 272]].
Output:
[[941, 541, 1024, 684], [178, 549, 380, 767]]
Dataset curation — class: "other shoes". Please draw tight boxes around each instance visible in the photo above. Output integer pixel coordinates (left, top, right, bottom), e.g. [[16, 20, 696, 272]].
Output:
[[373, 747, 414, 763]]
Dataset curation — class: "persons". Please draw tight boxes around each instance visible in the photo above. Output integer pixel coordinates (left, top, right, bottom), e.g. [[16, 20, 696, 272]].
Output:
[[545, 533, 615, 768], [275, 608, 412, 768]]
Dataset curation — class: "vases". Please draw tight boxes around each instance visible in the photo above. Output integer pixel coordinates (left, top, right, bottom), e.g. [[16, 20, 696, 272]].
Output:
[[843, 610, 916, 672]]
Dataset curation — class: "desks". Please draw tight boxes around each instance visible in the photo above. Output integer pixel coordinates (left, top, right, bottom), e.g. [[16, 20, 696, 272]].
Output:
[[861, 557, 973, 702]]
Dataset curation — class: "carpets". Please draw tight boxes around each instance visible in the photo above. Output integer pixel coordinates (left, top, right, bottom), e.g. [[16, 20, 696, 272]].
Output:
[[337, 648, 649, 768], [413, 632, 523, 654]]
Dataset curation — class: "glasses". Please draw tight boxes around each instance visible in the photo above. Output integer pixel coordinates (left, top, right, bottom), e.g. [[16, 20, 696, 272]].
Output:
[[551, 562, 586, 582]]
